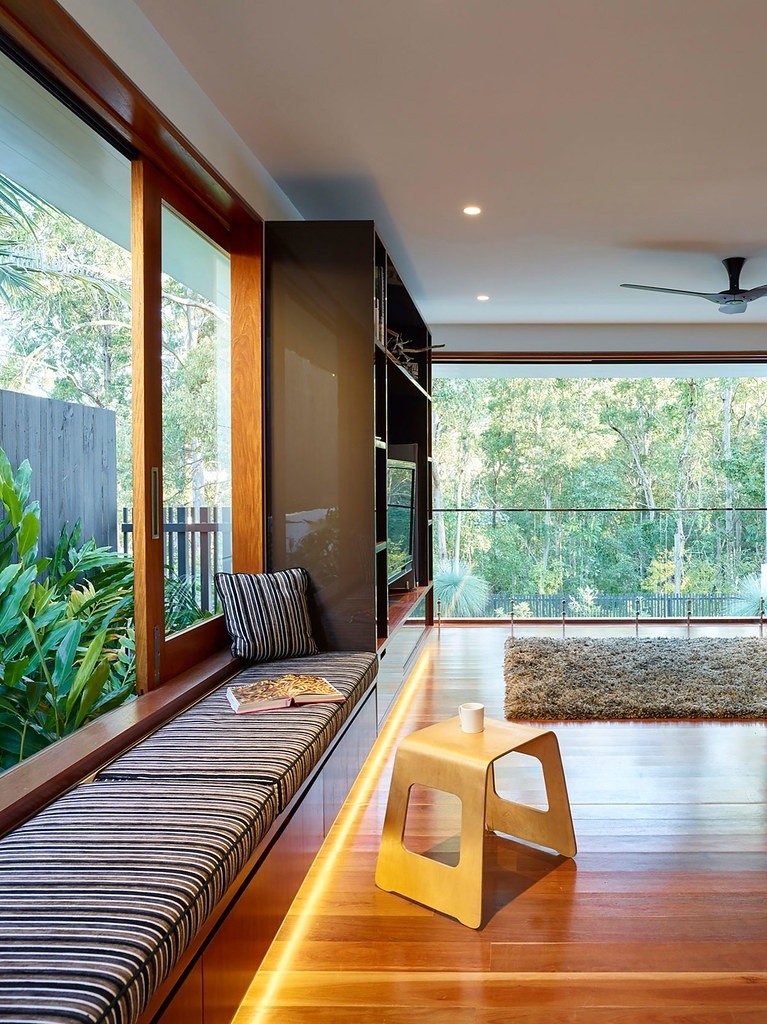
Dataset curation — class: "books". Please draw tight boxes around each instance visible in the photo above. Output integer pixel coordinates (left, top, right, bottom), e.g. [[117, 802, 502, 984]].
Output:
[[225, 674, 347, 715]]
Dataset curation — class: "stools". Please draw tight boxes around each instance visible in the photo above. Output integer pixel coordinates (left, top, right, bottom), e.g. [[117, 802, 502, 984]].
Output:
[[374, 713, 578, 931]]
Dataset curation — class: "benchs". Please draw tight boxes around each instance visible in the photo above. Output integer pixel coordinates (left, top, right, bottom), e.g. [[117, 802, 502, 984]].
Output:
[[0, 648, 377, 1024]]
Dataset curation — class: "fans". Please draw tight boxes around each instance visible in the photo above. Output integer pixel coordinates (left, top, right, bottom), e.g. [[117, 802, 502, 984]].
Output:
[[620, 256, 767, 314]]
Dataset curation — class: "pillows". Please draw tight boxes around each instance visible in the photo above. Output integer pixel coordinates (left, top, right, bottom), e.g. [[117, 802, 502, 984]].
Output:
[[215, 567, 320, 661]]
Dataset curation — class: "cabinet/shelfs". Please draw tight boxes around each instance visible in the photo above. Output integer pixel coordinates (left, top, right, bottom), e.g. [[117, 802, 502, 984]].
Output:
[[265, 217, 444, 652]]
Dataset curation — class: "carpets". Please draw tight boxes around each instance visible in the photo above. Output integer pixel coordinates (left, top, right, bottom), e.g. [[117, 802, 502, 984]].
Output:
[[505, 636, 766, 722]]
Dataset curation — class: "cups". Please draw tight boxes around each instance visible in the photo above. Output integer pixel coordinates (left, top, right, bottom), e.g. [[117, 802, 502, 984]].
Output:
[[458, 703, 484, 733]]
